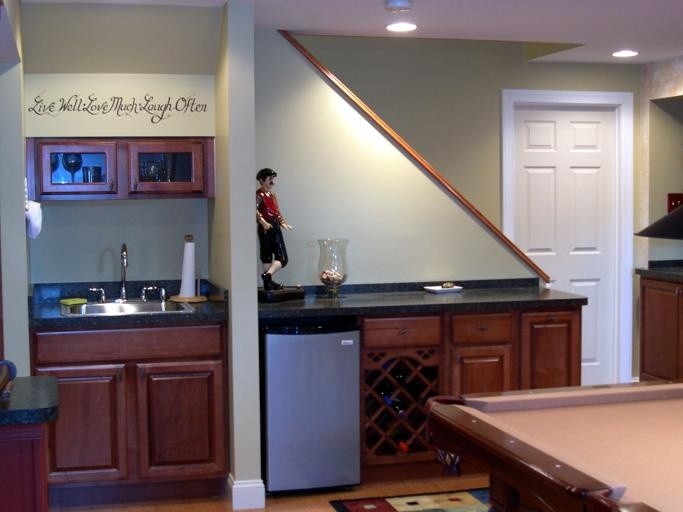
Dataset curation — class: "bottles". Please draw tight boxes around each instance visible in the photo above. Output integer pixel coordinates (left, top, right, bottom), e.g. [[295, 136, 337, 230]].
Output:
[[377, 372, 424, 453]]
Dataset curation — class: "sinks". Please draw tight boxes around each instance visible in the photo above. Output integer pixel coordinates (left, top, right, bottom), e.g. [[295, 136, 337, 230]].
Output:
[[64, 299, 189, 315]]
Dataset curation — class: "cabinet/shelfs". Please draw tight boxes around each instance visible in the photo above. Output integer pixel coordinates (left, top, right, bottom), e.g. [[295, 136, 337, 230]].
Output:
[[361, 305, 581, 482], [640, 279, 683, 381], [30, 322, 229, 507], [26, 137, 214, 201]]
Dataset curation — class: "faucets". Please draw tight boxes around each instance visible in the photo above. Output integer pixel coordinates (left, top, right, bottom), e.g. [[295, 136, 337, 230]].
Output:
[[119, 242, 127, 302]]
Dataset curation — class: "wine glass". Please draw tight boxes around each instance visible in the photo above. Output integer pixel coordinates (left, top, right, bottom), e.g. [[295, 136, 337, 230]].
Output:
[[315, 236, 350, 301], [62, 152, 83, 184]]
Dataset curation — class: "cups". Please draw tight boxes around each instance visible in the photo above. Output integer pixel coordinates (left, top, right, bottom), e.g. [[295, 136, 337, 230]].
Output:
[[82, 165, 103, 182]]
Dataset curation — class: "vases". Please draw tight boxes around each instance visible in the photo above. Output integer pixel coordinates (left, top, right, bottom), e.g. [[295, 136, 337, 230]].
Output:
[[318, 238, 348, 298]]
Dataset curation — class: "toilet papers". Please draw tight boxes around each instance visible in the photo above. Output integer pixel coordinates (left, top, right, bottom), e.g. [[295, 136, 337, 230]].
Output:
[[177, 242, 198, 298]]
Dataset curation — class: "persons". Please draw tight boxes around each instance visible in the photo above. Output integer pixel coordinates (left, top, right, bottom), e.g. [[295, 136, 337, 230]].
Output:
[[255, 169, 293, 290]]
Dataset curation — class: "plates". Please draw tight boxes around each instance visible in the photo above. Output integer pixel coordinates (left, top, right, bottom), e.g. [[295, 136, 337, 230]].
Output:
[[423, 284, 463, 294]]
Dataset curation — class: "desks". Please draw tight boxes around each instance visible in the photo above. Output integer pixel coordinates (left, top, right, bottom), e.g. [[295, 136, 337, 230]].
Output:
[[425, 380, 683, 512]]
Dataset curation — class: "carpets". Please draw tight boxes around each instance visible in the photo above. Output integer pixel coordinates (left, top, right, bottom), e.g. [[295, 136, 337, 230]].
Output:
[[329, 488, 491, 512]]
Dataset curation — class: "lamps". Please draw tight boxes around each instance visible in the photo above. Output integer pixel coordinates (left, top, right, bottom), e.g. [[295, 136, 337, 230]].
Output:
[[385, 0, 413, 13]]
